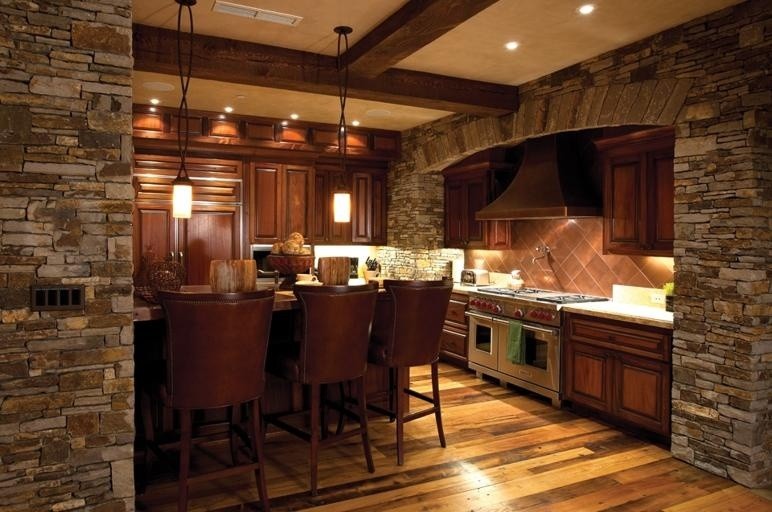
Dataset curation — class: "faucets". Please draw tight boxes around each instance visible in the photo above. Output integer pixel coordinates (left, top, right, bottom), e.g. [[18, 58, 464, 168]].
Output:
[[532, 245, 549, 262]]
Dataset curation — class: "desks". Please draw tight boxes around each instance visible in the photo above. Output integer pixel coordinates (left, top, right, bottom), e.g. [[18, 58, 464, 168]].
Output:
[[134, 282, 410, 448]]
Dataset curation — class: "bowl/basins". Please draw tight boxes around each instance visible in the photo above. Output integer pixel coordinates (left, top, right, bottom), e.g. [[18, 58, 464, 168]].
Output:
[[369, 277, 394, 288]]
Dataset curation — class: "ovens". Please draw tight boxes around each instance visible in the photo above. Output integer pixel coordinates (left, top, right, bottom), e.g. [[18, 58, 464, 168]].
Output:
[[464, 310, 560, 392]]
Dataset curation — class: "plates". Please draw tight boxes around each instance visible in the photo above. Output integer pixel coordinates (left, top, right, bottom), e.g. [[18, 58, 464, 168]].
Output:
[[294, 281, 324, 286]]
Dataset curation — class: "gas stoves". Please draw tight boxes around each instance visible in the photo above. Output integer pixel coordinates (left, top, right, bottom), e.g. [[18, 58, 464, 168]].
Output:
[[467, 286, 609, 305]]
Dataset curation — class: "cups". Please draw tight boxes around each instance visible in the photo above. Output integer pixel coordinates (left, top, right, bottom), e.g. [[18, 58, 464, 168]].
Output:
[[297, 273, 317, 283], [363, 270, 380, 284]]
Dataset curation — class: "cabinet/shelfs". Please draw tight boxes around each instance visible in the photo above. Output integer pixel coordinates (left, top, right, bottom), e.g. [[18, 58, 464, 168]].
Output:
[[603, 137, 674, 256], [247, 162, 387, 244], [564, 311, 672, 438], [445, 170, 511, 249], [134, 202, 244, 281]]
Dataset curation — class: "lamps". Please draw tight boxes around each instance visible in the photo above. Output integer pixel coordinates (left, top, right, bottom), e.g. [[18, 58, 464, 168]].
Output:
[[330, 25, 355, 227], [170, 0, 197, 220]]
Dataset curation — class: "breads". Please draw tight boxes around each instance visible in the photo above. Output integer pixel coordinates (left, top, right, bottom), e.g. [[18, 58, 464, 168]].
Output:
[[271, 232, 311, 256]]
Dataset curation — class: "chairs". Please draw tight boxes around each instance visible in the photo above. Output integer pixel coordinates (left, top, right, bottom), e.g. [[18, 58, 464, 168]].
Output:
[[336, 280, 453, 467], [253, 280, 379, 496], [153, 285, 275, 512]]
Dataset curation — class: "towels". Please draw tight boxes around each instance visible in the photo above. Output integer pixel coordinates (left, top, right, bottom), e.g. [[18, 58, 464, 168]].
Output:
[[503, 320, 526, 364]]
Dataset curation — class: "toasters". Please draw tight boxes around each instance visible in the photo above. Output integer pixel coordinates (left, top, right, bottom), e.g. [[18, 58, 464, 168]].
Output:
[[460, 268, 489, 287]]
